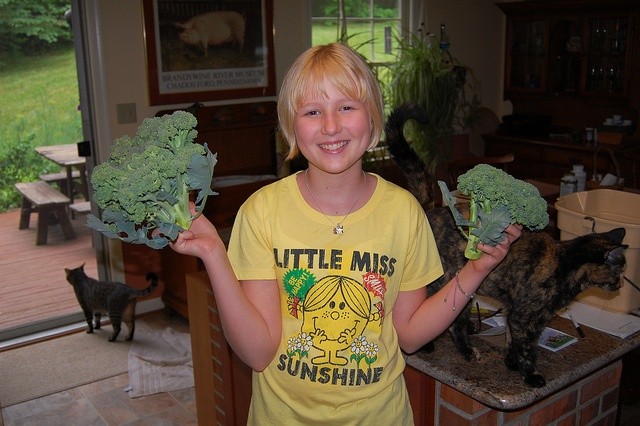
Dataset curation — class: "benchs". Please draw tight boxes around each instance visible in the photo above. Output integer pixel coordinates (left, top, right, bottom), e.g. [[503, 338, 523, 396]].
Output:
[[14, 181, 78, 246], [68, 202, 92, 220], [38, 170, 89, 183]]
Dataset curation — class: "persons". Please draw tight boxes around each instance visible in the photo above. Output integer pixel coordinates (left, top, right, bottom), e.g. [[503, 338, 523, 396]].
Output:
[[151, 41, 524, 426]]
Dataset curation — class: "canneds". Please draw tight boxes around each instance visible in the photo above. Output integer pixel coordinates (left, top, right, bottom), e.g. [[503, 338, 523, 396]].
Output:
[[586, 128, 593, 143]]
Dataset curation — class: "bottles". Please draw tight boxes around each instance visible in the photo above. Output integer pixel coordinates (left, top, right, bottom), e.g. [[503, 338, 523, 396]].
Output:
[[586, 127, 593, 141], [607, 68, 616, 92], [594, 128, 598, 142], [612, 23, 621, 51]]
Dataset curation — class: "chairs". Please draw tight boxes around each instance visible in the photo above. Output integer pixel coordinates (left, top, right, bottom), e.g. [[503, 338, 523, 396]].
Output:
[[446, 152, 515, 174]]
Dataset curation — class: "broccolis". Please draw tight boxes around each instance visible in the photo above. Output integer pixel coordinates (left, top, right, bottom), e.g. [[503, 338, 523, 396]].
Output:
[[83, 109, 218, 250], [438, 162, 550, 258]]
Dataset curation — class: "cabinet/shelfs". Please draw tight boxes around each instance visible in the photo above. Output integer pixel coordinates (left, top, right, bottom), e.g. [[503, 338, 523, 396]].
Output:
[[491, 128, 640, 225], [492, 0, 640, 130], [153, 101, 279, 316]]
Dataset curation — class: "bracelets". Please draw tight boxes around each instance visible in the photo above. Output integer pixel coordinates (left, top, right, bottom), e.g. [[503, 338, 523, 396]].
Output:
[[443, 270, 476, 311]]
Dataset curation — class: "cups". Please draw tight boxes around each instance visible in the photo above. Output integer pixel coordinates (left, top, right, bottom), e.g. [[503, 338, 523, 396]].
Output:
[[624, 120, 631, 123], [614, 114, 621, 120], [606, 118, 613, 123]]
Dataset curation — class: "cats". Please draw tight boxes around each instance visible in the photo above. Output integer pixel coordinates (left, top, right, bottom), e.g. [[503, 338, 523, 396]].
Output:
[[64, 261, 159, 341], [383, 103, 629, 387]]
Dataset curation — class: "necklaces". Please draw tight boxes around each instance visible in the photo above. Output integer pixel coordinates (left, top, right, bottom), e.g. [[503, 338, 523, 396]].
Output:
[[304, 166, 367, 235]]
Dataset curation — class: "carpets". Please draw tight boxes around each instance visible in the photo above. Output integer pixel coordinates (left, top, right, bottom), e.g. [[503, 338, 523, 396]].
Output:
[[124, 317, 194, 398]]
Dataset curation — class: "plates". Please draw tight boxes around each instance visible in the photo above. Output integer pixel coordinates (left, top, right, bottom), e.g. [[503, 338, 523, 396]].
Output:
[[603, 122, 614, 126], [613, 120, 622, 123], [622, 123, 632, 126]]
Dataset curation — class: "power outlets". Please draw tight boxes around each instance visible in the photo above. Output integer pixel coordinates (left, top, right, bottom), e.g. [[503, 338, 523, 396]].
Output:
[[115, 102, 138, 126]]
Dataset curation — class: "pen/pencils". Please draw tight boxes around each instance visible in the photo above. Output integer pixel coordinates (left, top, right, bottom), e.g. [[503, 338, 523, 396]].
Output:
[[565, 308, 585, 338]]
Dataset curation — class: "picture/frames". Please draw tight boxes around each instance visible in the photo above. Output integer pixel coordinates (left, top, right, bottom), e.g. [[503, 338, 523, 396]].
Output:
[[139, 0, 278, 107]]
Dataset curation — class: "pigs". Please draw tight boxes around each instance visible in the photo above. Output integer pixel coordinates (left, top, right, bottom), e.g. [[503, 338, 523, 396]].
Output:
[[174, 11, 246, 58]]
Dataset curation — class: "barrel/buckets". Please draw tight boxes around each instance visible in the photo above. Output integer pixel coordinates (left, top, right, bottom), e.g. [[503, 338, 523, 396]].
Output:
[[554, 189, 640, 313]]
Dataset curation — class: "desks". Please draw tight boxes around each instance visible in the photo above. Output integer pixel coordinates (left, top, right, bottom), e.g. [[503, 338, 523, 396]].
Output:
[[35, 143, 89, 220]]
